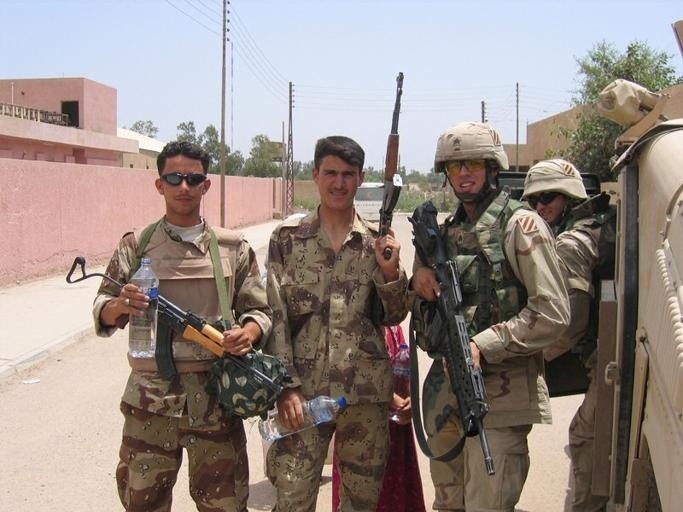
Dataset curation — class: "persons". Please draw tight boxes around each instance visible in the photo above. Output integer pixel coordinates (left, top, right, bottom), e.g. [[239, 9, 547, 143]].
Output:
[[261, 135, 409, 512], [91, 143, 273, 512], [520, 158, 617, 512], [405, 122, 572, 512], [330, 326, 426, 512]]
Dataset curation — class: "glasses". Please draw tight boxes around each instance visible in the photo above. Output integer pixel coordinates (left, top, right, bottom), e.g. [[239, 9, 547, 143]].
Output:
[[528, 192, 560, 210], [162, 172, 206, 185], [445, 160, 486, 176]]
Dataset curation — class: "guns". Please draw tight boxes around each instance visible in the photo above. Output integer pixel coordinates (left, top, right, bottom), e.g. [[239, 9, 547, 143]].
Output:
[[407, 200, 495, 475], [66, 256, 283, 396], [370, 71, 404, 326]]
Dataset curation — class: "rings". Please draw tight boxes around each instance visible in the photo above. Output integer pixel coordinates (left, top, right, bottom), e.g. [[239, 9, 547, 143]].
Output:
[[125, 298, 130, 306]]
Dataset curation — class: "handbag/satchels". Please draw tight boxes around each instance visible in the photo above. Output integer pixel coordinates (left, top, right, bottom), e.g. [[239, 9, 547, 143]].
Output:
[[207, 347, 286, 418]]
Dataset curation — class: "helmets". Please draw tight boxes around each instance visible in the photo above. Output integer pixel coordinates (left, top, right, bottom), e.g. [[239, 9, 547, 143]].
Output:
[[522, 159, 588, 199], [435, 122, 510, 173]]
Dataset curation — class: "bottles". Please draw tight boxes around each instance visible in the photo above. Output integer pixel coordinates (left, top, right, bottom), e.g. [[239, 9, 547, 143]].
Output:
[[257, 395, 346, 442], [127, 257, 159, 359], [392, 344, 411, 394]]
